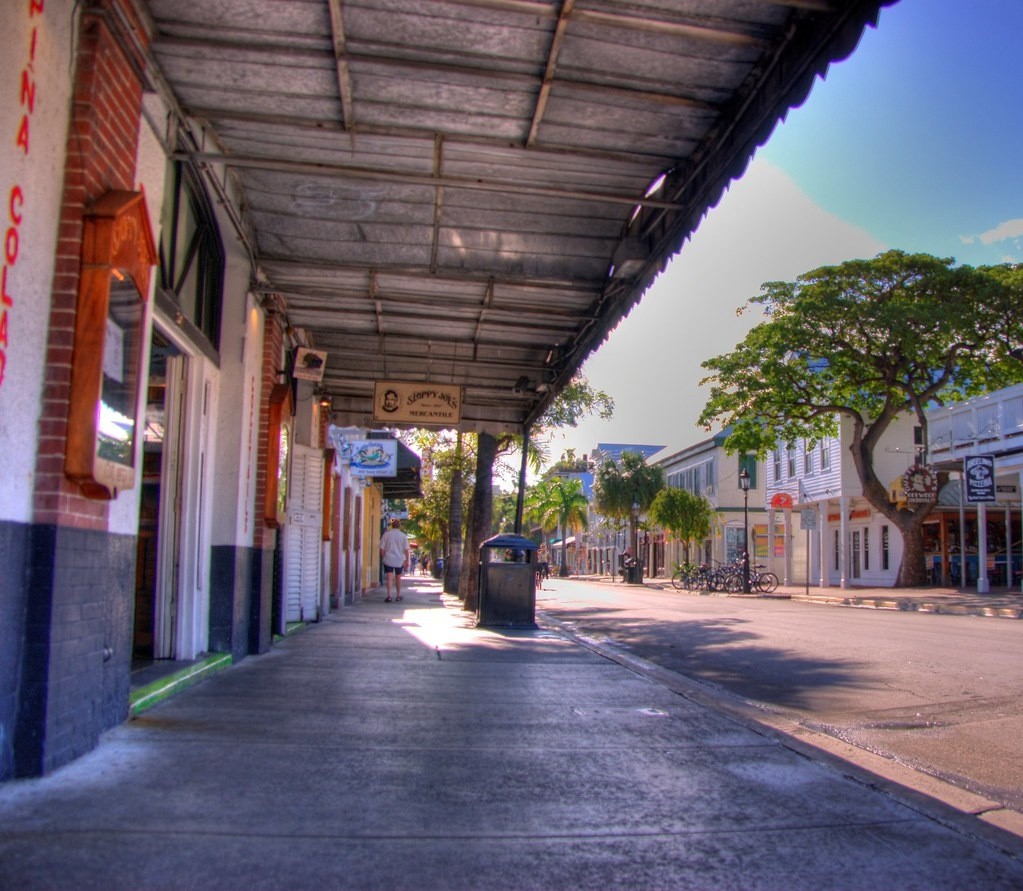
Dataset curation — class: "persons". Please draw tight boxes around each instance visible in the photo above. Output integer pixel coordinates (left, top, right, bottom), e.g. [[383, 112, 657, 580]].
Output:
[[539, 543, 550, 582], [409, 551, 430, 574], [379, 520, 410, 602]]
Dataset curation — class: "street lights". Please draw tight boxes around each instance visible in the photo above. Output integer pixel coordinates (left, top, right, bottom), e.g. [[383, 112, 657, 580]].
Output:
[[738, 467, 752, 594], [631, 501, 641, 583]]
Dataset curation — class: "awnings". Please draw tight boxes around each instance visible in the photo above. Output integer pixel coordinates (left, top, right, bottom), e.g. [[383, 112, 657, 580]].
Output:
[[366, 433, 425, 500]]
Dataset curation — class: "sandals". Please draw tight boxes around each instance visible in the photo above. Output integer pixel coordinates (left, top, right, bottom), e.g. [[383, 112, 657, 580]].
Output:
[[396, 596, 403, 602], [386, 596, 392, 602]]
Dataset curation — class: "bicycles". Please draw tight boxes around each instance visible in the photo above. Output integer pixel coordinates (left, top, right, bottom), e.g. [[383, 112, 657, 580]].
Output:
[[672, 554, 779, 595]]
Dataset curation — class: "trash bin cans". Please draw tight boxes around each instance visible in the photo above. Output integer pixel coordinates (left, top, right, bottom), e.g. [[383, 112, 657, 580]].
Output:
[[477, 533, 542, 631], [624, 557, 645, 584]]
